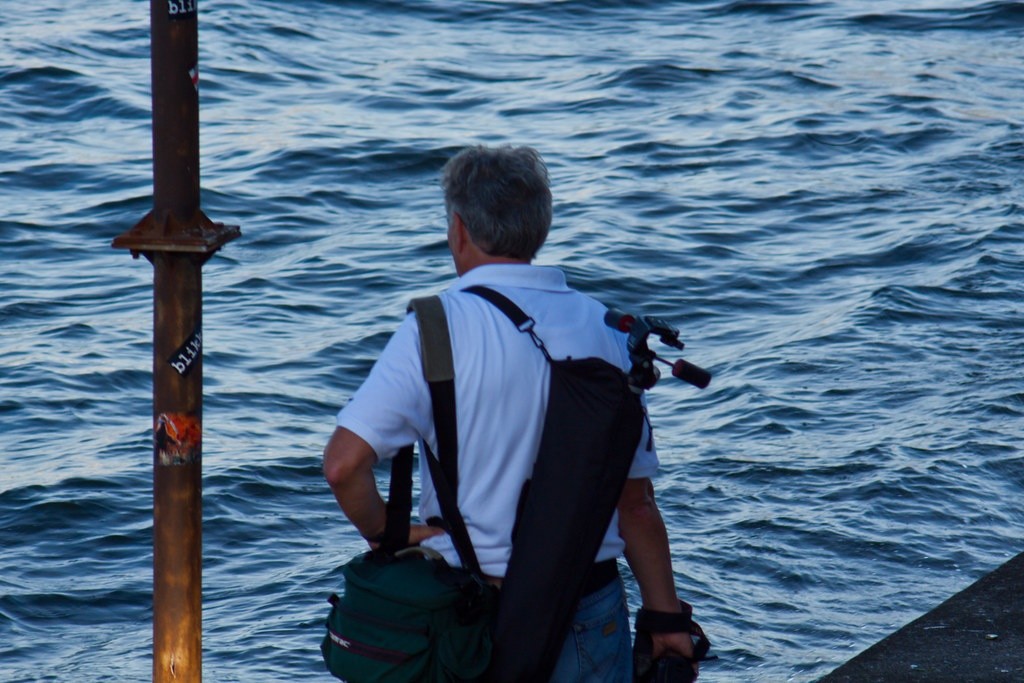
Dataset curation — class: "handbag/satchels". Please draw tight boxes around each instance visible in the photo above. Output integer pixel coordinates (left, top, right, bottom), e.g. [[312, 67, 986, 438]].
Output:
[[320, 355, 644, 683]]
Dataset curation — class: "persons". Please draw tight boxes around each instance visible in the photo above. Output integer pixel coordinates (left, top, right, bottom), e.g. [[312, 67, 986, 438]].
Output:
[[322, 147, 708, 683]]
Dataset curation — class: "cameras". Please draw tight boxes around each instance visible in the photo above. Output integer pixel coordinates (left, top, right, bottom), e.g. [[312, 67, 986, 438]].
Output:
[[635, 648, 698, 682]]
